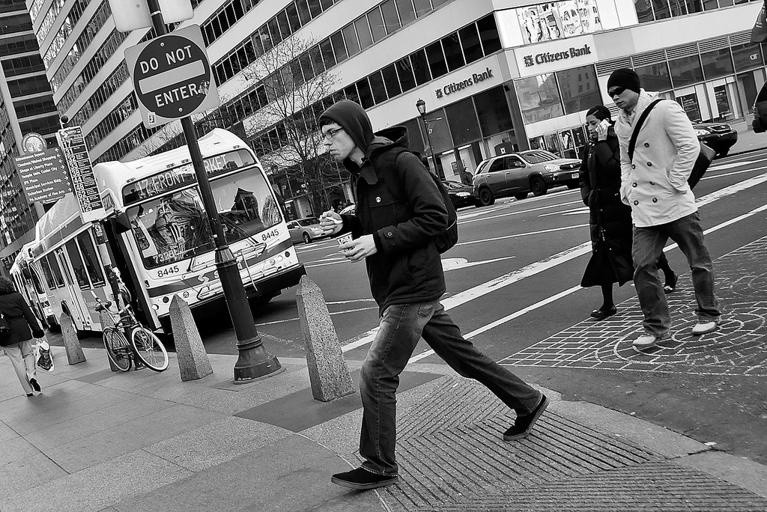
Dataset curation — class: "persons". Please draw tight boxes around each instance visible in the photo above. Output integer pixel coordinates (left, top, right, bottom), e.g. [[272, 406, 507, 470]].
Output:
[[607, 69, 722, 348], [579, 105, 678, 318], [0, 277, 44, 395], [319, 99, 549, 490], [462, 166, 473, 185], [330, 199, 354, 214]]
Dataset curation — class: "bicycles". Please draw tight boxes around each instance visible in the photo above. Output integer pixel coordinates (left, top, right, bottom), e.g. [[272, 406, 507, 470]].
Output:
[[89, 267, 170, 373]]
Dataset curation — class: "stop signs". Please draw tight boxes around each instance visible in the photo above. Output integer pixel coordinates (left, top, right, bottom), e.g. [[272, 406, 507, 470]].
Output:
[[123, 23, 221, 130]]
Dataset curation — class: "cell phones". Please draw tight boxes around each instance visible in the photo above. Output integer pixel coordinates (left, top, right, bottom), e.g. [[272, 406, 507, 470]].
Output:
[[595, 118, 612, 133]]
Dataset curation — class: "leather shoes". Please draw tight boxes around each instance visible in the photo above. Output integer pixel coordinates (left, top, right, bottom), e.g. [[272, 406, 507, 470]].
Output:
[[590, 304, 618, 319], [663, 270, 678, 294]]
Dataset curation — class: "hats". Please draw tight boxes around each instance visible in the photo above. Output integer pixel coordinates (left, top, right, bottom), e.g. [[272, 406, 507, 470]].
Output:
[[607, 68, 641, 95]]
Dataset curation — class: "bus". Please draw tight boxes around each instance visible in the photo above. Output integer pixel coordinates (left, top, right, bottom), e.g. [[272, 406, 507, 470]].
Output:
[[29, 128, 308, 342], [8, 239, 58, 335]]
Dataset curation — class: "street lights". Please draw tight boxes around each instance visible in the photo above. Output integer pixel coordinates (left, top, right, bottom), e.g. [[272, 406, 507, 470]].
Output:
[[416, 98, 442, 182]]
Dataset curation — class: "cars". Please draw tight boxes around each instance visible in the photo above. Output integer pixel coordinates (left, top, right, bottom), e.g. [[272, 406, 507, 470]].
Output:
[[440, 181, 476, 209], [750, 80, 767, 133], [339, 205, 355, 216], [471, 150, 583, 206], [690, 121, 738, 158]]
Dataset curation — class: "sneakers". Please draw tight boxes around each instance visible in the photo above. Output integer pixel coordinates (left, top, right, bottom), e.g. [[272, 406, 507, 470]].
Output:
[[502, 392, 550, 441], [632, 327, 672, 350], [29, 378, 41, 392], [330, 466, 399, 491], [26, 393, 34, 397], [691, 317, 720, 336]]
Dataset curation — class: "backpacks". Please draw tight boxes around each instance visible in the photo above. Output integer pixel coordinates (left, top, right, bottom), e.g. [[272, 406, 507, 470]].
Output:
[[380, 146, 458, 255]]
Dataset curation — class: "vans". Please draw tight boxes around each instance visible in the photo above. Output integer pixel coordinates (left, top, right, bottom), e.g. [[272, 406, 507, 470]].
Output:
[[287, 217, 323, 245]]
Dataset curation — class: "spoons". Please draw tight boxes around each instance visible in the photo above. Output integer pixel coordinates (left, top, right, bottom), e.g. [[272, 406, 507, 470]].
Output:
[[325, 216, 343, 225]]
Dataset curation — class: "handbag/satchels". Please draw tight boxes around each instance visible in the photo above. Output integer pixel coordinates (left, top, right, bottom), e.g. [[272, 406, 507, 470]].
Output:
[[686, 142, 717, 191], [0, 312, 11, 334]]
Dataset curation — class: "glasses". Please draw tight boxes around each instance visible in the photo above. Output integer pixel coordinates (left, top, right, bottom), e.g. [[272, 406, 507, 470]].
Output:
[[318, 127, 344, 144], [608, 87, 627, 98]]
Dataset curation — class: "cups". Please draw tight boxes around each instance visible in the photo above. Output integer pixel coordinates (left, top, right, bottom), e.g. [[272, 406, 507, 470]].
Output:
[[337, 232, 357, 260]]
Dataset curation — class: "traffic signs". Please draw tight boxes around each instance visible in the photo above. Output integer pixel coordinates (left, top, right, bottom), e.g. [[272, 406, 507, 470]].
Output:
[[12, 146, 73, 205]]
